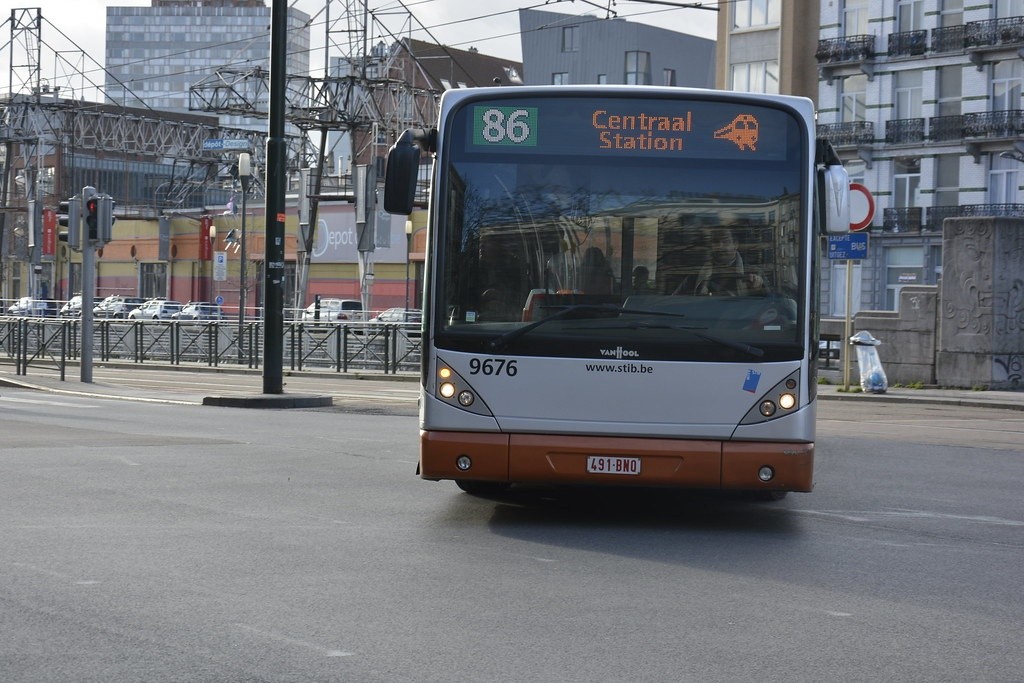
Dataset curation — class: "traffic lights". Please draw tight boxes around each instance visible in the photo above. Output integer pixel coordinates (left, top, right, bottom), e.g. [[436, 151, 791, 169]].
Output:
[[59, 197, 79, 249], [87, 196, 102, 242], [104, 196, 116, 243]]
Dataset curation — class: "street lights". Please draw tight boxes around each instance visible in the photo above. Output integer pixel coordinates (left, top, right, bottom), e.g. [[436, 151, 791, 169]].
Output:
[[238, 152, 251, 365], [404, 221, 413, 322], [208, 224, 215, 316]]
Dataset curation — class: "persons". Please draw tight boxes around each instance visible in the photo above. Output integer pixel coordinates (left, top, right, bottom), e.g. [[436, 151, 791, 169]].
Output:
[[672, 226, 774, 296], [449, 228, 656, 322]]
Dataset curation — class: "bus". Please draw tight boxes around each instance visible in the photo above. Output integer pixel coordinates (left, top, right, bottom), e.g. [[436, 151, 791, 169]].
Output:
[[382, 82, 854, 508]]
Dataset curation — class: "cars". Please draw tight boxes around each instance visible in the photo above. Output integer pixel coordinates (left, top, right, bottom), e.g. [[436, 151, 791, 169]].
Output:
[[93, 295, 147, 319], [127, 300, 183, 320], [170, 304, 223, 321], [7, 297, 57, 320], [59, 296, 105, 319], [368, 308, 423, 336]]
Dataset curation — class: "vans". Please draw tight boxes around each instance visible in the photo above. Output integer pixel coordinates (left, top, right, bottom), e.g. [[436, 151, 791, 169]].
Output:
[[301, 297, 367, 334]]
